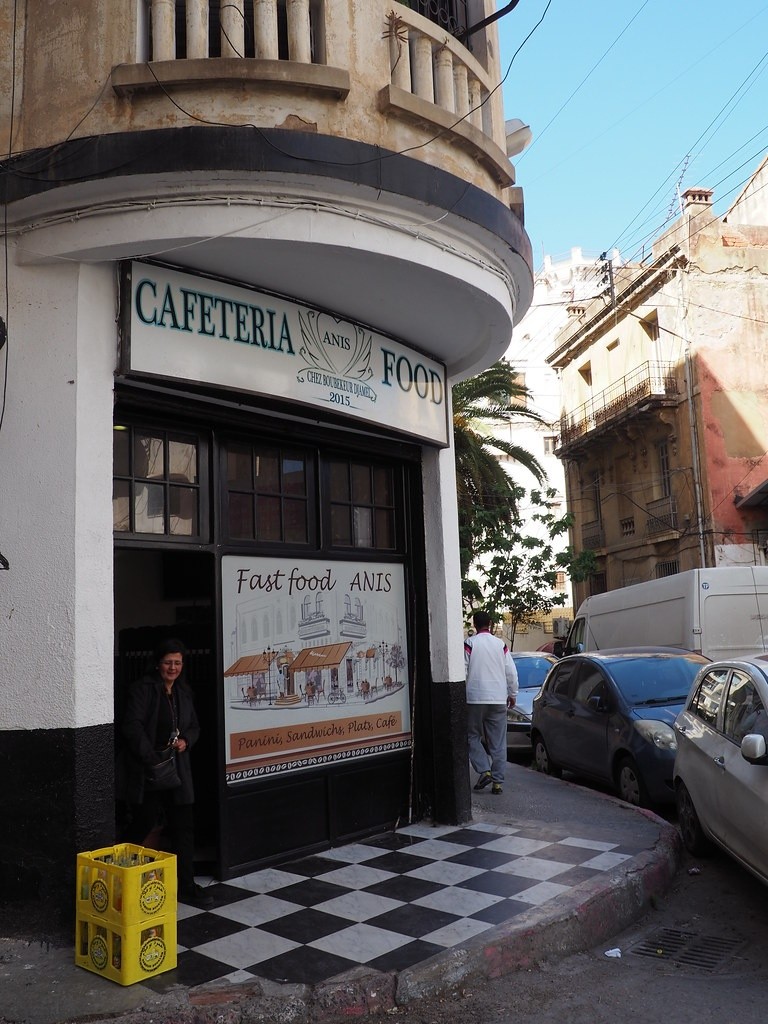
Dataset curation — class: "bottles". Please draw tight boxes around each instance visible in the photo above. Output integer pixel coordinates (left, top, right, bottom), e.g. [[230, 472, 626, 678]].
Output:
[[80, 921, 162, 972], [81, 850, 164, 913]]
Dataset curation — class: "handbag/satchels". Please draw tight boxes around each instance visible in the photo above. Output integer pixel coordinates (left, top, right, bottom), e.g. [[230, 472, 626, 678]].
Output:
[[144, 745, 183, 792]]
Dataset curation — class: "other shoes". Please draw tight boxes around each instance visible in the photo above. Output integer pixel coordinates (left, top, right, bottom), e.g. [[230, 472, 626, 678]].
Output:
[[178, 883, 214, 905]]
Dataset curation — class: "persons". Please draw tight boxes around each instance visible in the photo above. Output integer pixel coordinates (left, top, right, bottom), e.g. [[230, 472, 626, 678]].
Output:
[[127, 643, 215, 903], [464, 611, 519, 794]]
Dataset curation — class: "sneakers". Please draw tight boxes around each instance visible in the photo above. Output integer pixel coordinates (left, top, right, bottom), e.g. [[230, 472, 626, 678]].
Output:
[[474, 770, 492, 789], [492, 782, 504, 794]]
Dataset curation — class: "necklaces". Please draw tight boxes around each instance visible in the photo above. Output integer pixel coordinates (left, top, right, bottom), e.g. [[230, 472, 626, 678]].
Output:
[[166, 692, 177, 731]]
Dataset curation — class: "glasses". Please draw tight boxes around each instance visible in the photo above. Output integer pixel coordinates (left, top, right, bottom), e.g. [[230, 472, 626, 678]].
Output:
[[161, 660, 184, 666]]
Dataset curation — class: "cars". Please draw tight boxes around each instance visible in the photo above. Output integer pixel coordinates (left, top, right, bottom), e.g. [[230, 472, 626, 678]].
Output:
[[481, 652, 561, 765], [531, 644, 714, 815], [672, 655, 768, 886]]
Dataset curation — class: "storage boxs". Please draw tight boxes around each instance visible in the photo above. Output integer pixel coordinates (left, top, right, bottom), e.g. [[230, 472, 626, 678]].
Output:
[[74, 843, 179, 987]]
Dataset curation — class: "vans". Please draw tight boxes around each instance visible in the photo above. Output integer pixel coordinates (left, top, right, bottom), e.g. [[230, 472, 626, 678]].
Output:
[[551, 565, 768, 662]]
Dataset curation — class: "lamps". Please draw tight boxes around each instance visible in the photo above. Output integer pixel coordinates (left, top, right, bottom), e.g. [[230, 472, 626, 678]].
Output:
[[504, 119, 534, 156]]
